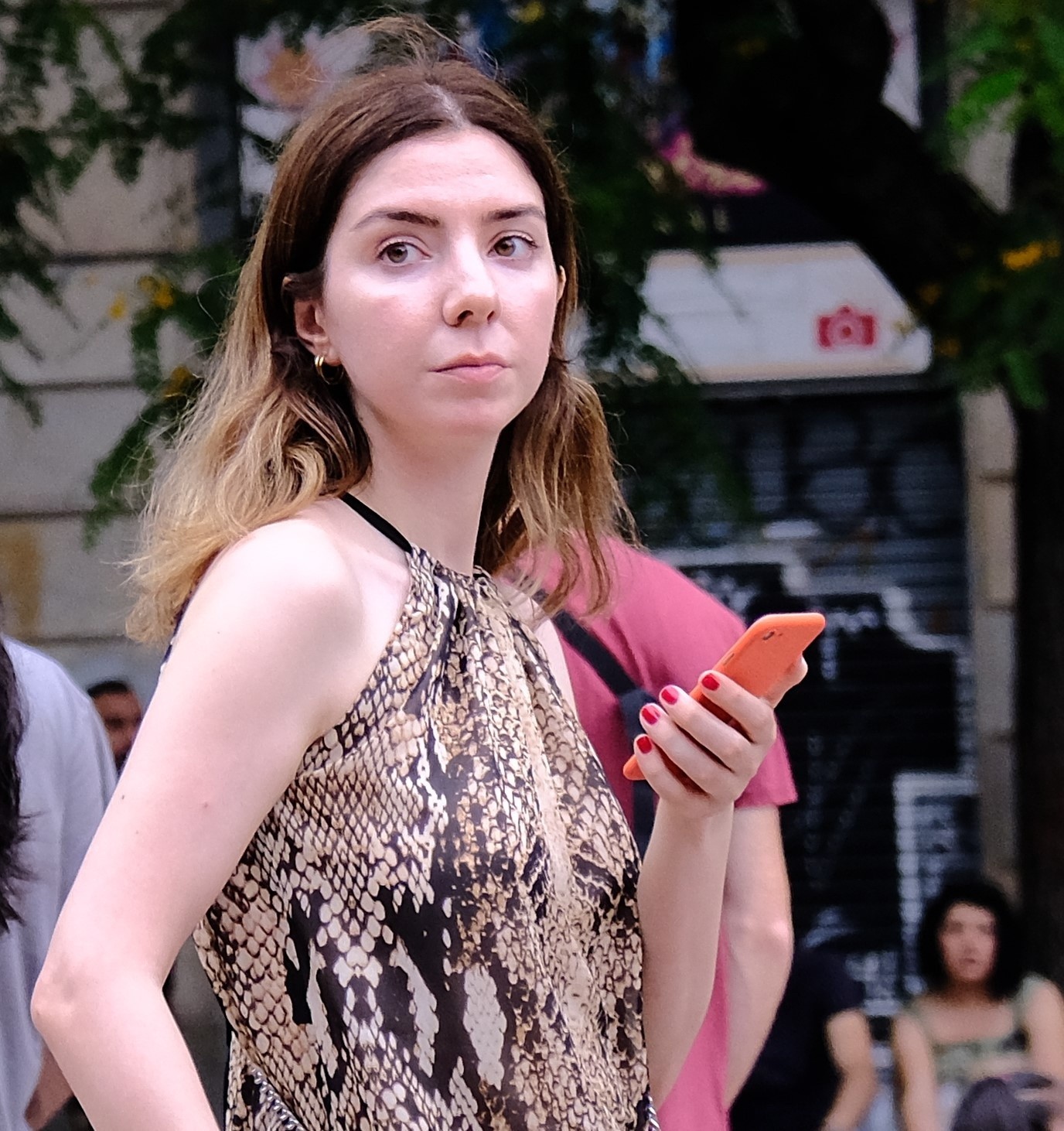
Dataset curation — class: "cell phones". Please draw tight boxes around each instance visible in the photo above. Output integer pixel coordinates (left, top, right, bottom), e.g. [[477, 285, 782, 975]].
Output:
[[623, 613, 825, 782]]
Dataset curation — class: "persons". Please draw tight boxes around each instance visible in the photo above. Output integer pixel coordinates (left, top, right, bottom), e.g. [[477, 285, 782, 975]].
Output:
[[476, 522, 801, 1131], [888, 863, 1061, 1131], [724, 928, 876, 1131], [0, 626, 232, 1131], [31, 15, 807, 1131]]
